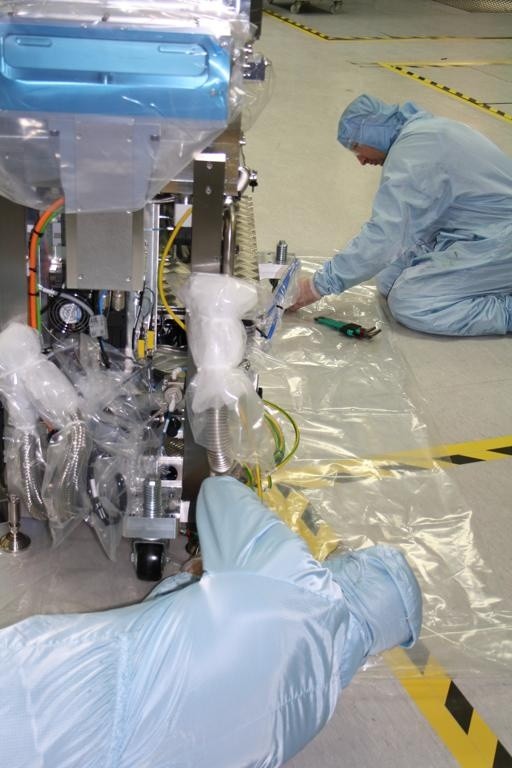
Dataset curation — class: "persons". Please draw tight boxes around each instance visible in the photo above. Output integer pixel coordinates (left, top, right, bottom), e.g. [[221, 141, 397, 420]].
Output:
[[0, 475, 422, 768], [288, 93, 512, 336]]
